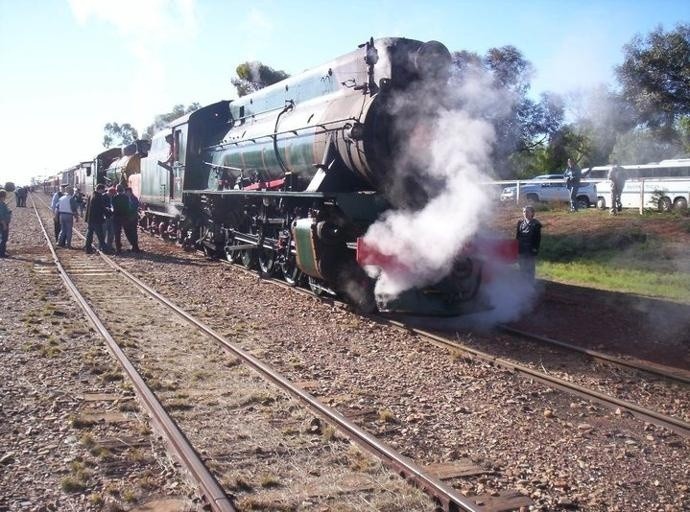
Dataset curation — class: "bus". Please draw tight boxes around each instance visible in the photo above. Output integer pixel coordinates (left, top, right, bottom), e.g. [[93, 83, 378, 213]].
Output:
[[579, 158, 690, 212]]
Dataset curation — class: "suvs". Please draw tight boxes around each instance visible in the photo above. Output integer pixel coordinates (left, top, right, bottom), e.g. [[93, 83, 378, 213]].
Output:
[[501, 168, 602, 210]]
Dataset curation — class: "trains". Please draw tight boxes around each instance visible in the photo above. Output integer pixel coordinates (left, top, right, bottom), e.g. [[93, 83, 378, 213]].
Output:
[[38, 34, 521, 319]]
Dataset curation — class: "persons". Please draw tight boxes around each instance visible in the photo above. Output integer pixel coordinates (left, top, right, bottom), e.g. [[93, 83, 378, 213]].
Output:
[[50, 179, 142, 255], [607, 159, 629, 216], [563, 157, 582, 212], [163, 134, 175, 164], [13, 186, 29, 207], [516, 205, 543, 281], [0, 189, 13, 257]]
